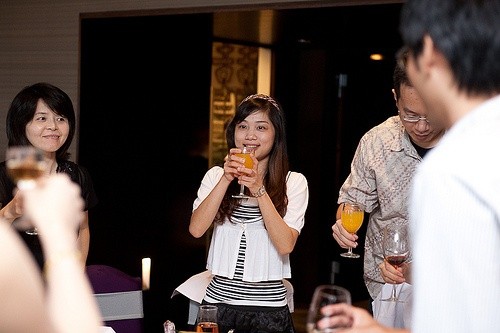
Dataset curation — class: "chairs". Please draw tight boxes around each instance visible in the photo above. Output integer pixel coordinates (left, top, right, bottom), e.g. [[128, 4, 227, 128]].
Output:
[[86, 264, 142, 333], [173, 270, 295, 333]]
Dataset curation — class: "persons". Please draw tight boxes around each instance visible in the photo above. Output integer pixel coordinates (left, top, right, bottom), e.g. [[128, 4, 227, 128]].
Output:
[[190, 94, 309, 333], [318, 0, 500, 333], [0, 82, 105, 333]]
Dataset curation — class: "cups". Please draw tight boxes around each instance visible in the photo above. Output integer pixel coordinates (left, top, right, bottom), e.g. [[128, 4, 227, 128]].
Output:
[[307, 285, 351, 333], [194, 305, 219, 333]]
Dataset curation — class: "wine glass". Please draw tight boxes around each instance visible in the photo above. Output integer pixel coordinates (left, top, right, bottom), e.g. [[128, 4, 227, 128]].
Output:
[[3, 145, 56, 235], [379, 224, 409, 301], [231, 147, 256, 199], [340, 203, 364, 258]]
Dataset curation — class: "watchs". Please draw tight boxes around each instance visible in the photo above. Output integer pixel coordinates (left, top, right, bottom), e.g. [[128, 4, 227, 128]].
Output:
[[251, 186, 265, 197]]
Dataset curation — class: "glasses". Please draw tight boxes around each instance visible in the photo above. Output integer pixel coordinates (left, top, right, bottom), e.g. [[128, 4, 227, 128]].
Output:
[[394, 43, 412, 71], [397, 98, 430, 123]]
